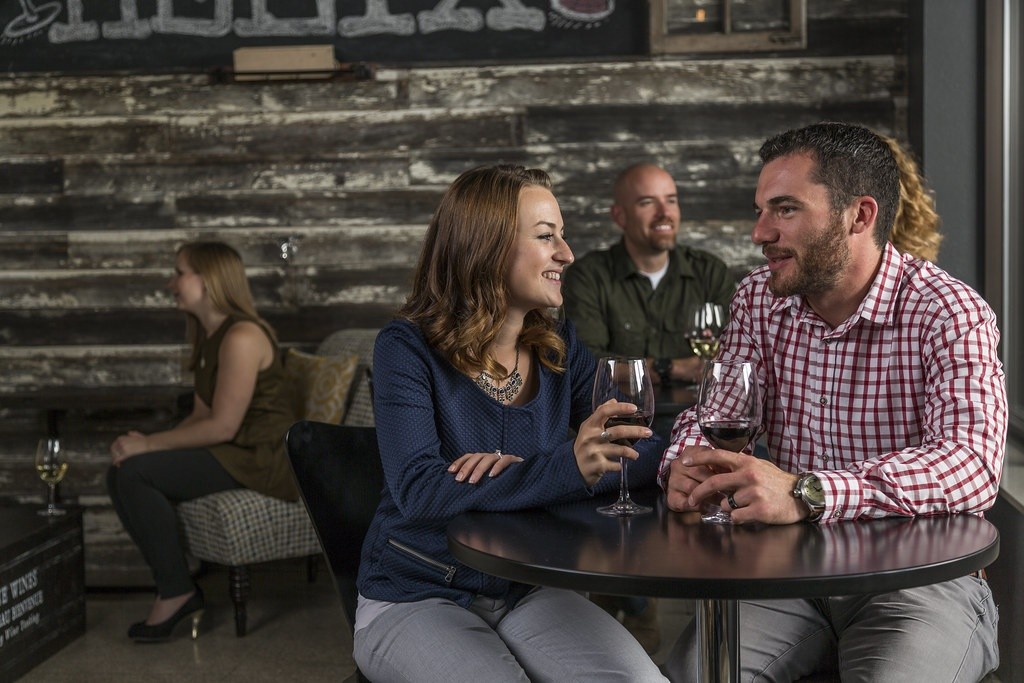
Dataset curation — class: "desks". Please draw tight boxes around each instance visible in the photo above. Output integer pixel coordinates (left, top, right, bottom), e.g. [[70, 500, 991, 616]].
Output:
[[448, 491, 1001, 683], [617, 380, 702, 414], [1, 500, 87, 683]]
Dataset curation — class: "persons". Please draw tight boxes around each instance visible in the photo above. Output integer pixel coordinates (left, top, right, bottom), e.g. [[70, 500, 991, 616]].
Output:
[[659, 121, 1009, 683], [353, 165, 668, 683], [106, 243, 301, 643], [561, 163, 738, 402]]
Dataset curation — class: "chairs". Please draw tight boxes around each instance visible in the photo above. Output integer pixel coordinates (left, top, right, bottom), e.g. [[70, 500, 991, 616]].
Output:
[[173, 329, 382, 636], [285, 421, 386, 683]]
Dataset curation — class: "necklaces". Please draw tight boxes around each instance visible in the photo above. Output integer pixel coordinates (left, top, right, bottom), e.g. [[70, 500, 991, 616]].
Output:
[[471, 347, 523, 403], [200, 344, 209, 370]]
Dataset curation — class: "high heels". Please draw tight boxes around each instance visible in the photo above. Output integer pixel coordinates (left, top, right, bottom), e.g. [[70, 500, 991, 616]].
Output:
[[127, 582, 205, 641]]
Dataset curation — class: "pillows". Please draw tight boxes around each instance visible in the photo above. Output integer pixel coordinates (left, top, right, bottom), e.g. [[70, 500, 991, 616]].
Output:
[[284, 346, 360, 426]]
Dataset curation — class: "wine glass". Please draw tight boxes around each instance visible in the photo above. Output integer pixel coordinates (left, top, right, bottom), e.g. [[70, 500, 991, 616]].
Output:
[[685, 304, 721, 367], [36, 438, 69, 518], [697, 359, 763, 524], [591, 356, 656, 514]]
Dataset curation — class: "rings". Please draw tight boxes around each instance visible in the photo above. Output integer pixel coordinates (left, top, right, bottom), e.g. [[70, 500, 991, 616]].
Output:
[[494, 450, 503, 459], [115, 451, 120, 456], [601, 429, 610, 441], [727, 495, 738, 509]]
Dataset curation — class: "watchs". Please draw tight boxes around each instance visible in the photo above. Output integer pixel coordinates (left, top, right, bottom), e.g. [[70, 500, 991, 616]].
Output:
[[793, 472, 826, 523], [652, 357, 673, 383]]
